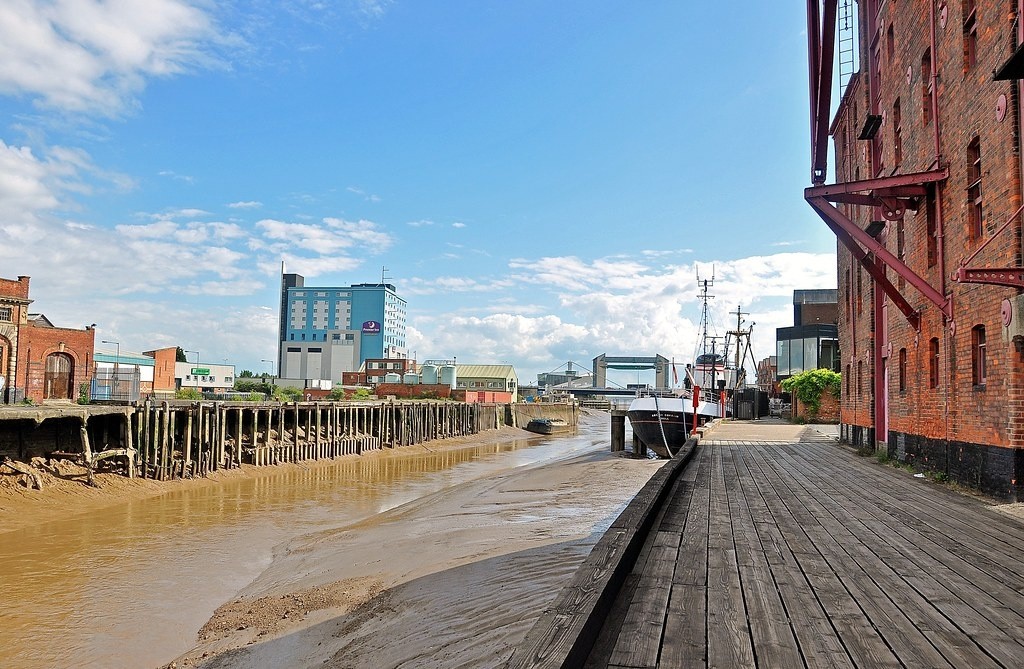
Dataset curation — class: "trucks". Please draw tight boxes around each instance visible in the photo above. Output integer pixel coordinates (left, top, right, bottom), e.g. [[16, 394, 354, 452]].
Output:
[[627, 384, 655, 390]]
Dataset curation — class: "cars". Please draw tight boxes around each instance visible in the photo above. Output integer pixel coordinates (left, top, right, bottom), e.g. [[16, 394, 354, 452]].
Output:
[[606, 387, 614, 390]]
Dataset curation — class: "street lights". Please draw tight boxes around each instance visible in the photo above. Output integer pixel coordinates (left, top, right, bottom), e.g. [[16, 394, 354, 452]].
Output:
[[102, 341, 119, 371], [185, 350, 199, 388], [262, 359, 273, 395]]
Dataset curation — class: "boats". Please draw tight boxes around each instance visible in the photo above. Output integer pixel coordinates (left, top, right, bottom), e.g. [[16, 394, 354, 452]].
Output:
[[627, 264, 746, 458]]
